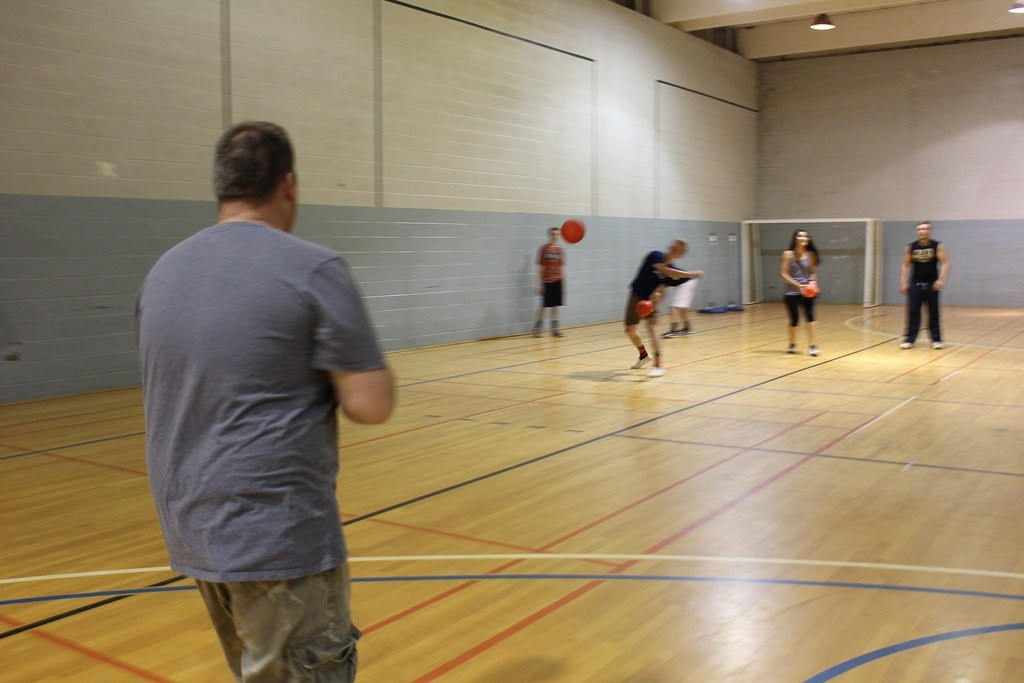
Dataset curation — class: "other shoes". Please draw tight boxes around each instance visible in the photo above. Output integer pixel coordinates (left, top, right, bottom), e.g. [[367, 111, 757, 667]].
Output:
[[931, 341, 944, 349], [809, 343, 819, 356], [662, 328, 693, 339], [533, 327, 540, 338], [647, 367, 663, 376], [631, 354, 652, 369], [550, 329, 563, 337], [899, 342, 912, 349], [787, 342, 796, 353]]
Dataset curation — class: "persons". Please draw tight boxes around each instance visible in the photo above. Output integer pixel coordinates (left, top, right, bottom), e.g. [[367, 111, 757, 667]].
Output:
[[533, 227, 565, 338], [661, 267, 698, 339], [899, 221, 952, 350], [779, 229, 823, 356], [134, 123, 397, 683], [623, 239, 703, 377]]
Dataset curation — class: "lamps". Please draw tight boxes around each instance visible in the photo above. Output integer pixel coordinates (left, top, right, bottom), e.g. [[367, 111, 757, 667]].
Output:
[[1008, 3, 1024, 13], [810, 14, 835, 31]]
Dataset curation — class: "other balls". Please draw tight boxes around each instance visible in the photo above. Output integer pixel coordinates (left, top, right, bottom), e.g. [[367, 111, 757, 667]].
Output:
[[560, 219, 586, 244], [802, 284, 817, 298], [636, 300, 653, 315]]
